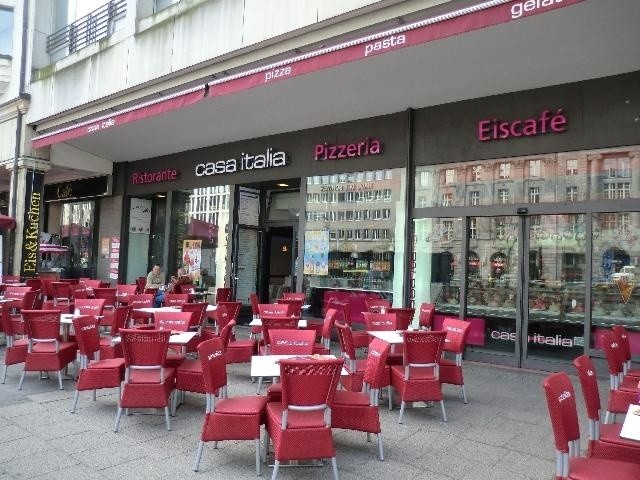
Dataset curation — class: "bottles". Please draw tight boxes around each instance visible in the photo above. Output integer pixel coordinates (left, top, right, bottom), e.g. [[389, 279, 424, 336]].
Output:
[[304, 253, 392, 293]]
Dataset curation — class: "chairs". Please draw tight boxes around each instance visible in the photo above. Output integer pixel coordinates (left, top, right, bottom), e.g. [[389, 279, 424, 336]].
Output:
[[2, 274, 471, 478], [540, 324, 638, 476]]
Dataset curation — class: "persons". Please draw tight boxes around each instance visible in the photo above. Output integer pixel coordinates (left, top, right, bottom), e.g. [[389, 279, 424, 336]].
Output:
[[145, 263, 163, 289], [153, 268, 191, 308]]
